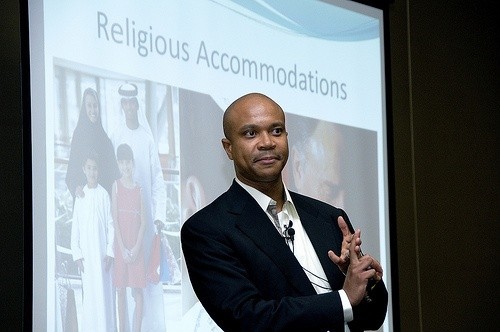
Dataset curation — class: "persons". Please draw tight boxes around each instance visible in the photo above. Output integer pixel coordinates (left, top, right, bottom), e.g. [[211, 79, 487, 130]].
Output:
[[70, 155, 117, 330], [110, 143, 161, 332], [181, 92, 389, 332], [112, 85, 169, 236], [181, 90, 236, 220], [290, 121, 361, 223], [65, 88, 120, 198]]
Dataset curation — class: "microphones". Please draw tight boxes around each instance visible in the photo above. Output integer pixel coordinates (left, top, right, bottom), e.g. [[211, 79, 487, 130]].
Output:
[[284, 220, 295, 243]]
